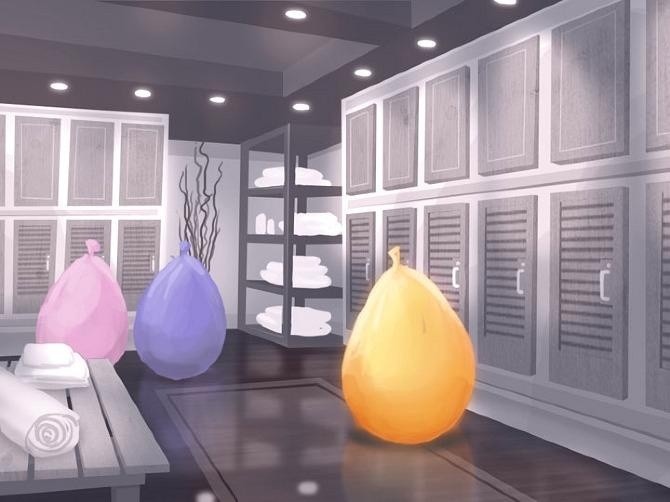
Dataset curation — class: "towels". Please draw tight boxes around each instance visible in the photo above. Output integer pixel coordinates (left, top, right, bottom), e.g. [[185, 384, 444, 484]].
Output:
[[256, 211, 344, 237], [256, 304, 332, 336], [15, 341, 89, 388], [259, 253, 332, 289], [0, 369, 81, 458], [253, 165, 333, 186]]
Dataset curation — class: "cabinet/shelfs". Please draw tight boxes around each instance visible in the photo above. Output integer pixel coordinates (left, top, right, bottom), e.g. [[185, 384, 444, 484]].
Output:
[[236, 122, 345, 351]]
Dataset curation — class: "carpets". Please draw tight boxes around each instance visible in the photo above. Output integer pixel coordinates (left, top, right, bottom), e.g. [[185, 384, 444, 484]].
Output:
[[154, 376, 540, 501]]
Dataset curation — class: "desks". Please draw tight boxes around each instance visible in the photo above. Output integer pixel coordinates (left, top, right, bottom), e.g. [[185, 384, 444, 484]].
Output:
[[0, 358, 171, 502]]
